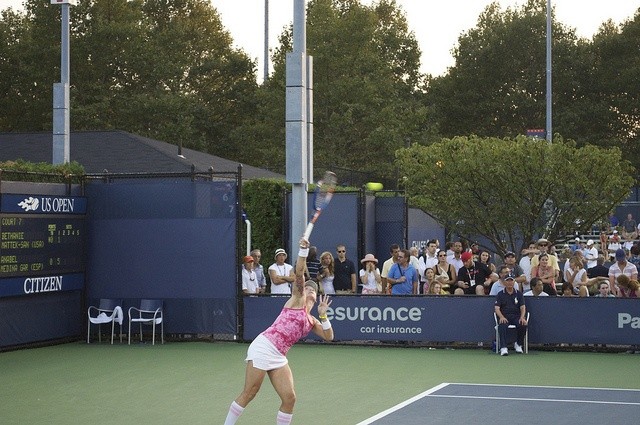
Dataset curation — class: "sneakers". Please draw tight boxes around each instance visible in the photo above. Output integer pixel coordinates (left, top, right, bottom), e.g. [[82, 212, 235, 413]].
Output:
[[514, 342, 522, 352], [501, 348, 508, 356]]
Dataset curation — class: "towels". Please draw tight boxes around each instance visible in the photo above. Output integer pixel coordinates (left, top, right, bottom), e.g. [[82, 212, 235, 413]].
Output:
[[98, 306, 123, 326]]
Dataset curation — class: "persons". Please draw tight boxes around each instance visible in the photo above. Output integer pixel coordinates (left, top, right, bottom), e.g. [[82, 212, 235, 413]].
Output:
[[616, 274, 640, 298], [587, 254, 609, 280], [381, 242, 400, 294], [489, 265, 518, 295], [495, 273, 530, 357], [479, 249, 496, 274], [224, 235, 333, 424], [445, 240, 463, 273], [241, 254, 260, 297], [470, 242, 483, 267], [530, 238, 559, 280], [422, 266, 436, 292], [386, 249, 419, 295], [518, 241, 535, 291], [410, 246, 419, 294], [560, 281, 573, 296], [446, 242, 452, 256], [596, 281, 613, 298], [432, 250, 458, 295], [320, 252, 335, 294], [564, 251, 583, 280], [565, 256, 588, 297], [333, 244, 358, 294], [294, 246, 328, 292], [522, 277, 548, 296], [359, 253, 381, 295], [269, 248, 295, 295], [455, 252, 498, 296], [240, 248, 267, 296], [427, 280, 441, 296], [501, 252, 527, 295], [596, 212, 640, 259], [531, 252, 556, 296], [607, 247, 637, 297], [571, 236, 585, 252], [584, 238, 599, 272], [419, 240, 438, 294]]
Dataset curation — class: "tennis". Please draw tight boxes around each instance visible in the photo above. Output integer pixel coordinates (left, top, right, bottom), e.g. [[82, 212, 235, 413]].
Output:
[[368, 182, 383, 192]]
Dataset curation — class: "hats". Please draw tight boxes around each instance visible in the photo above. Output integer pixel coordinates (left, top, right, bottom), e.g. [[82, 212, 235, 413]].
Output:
[[587, 239, 594, 245], [575, 237, 579, 241], [304, 279, 317, 294], [274, 249, 287, 260], [613, 230, 617, 233], [504, 274, 515, 281], [460, 252, 473, 260], [615, 249, 626, 261], [536, 239, 550, 245], [244, 256, 254, 263], [503, 251, 516, 258], [361, 254, 378, 263]]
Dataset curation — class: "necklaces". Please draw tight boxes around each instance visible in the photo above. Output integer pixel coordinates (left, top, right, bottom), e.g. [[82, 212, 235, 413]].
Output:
[[249, 271, 255, 281], [278, 266, 286, 276]]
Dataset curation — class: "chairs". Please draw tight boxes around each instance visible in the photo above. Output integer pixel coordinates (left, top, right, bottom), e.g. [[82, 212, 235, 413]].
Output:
[[127, 298, 164, 345], [555, 231, 640, 242], [493, 312, 531, 354], [86, 297, 123, 345]]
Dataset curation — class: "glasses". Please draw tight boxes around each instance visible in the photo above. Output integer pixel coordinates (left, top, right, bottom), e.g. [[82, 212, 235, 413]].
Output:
[[338, 250, 346, 253], [540, 244, 547, 247], [439, 255, 446, 257]]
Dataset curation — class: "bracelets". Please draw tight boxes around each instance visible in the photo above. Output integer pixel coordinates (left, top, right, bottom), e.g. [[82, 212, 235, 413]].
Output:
[[318, 274, 325, 279], [352, 291, 356, 293], [316, 313, 330, 322], [320, 320, 331, 331], [299, 248, 310, 257]]
